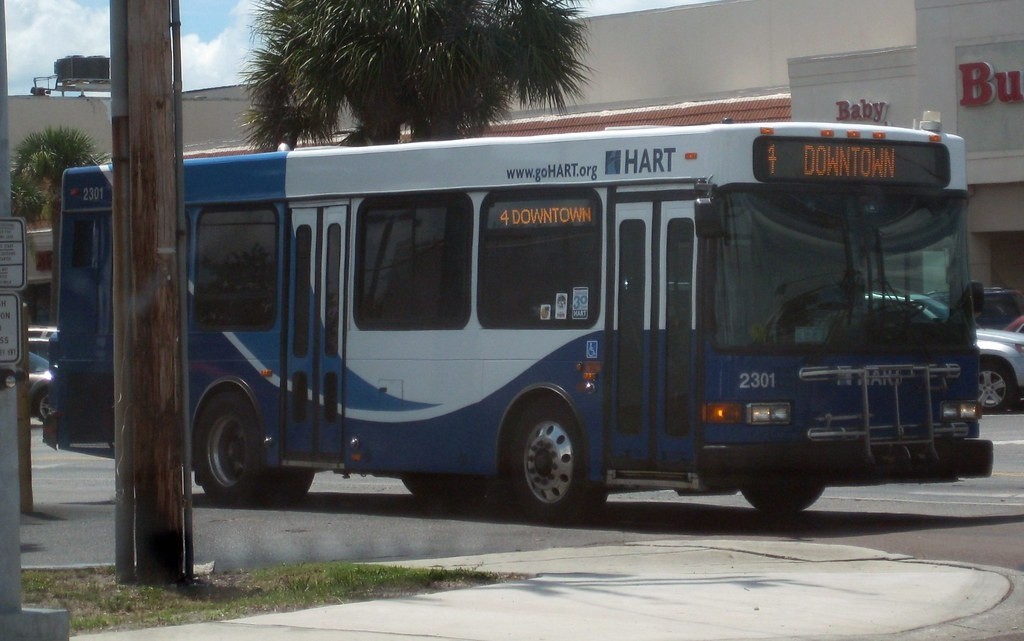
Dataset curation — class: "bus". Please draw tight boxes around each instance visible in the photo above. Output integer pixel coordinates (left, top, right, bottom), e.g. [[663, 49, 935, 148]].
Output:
[[43, 111, 993, 526]]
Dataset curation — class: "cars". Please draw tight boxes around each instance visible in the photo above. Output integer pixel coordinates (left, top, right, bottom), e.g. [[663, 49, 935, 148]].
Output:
[[865, 288, 1024, 414], [28, 352, 51, 421], [928, 287, 1024, 332]]
